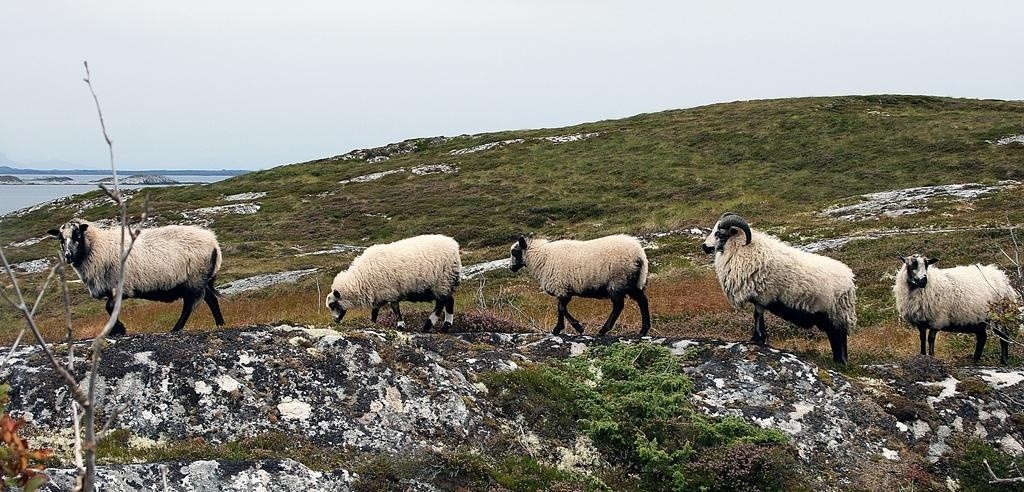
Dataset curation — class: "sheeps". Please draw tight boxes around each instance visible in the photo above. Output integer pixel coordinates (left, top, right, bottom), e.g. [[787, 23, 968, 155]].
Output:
[[507, 232, 651, 336], [46, 216, 226, 336], [323, 233, 463, 334], [701, 210, 860, 368], [890, 251, 1018, 366]]
